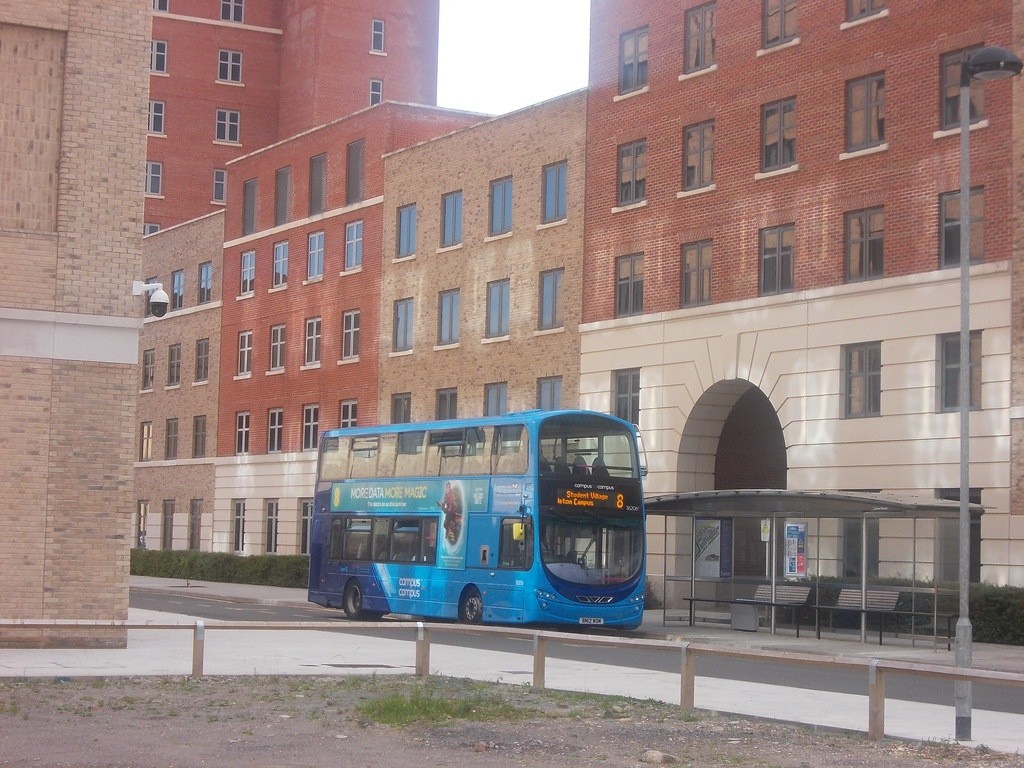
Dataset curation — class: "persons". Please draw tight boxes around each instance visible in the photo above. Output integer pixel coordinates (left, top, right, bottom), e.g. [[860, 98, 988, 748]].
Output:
[[520, 540, 533, 560], [436, 482, 462, 538], [555, 455, 609, 476]]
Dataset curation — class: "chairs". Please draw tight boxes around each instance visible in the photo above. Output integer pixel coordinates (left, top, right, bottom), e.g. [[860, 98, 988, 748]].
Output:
[[327, 453, 612, 476], [346, 541, 435, 563]]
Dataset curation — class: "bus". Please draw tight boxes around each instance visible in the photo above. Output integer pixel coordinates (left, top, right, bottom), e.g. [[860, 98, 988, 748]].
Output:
[[307, 408, 648, 630]]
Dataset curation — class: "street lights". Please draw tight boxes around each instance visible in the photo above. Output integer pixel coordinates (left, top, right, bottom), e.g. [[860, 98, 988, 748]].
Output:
[[955, 45, 1023, 741]]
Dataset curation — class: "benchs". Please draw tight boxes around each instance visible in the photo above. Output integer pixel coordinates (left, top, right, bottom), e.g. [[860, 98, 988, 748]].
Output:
[[831, 588, 901, 636], [737, 584, 812, 625]]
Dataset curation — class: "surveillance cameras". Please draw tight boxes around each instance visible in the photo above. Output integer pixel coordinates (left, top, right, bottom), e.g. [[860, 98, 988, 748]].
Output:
[[150, 290, 170, 317]]
